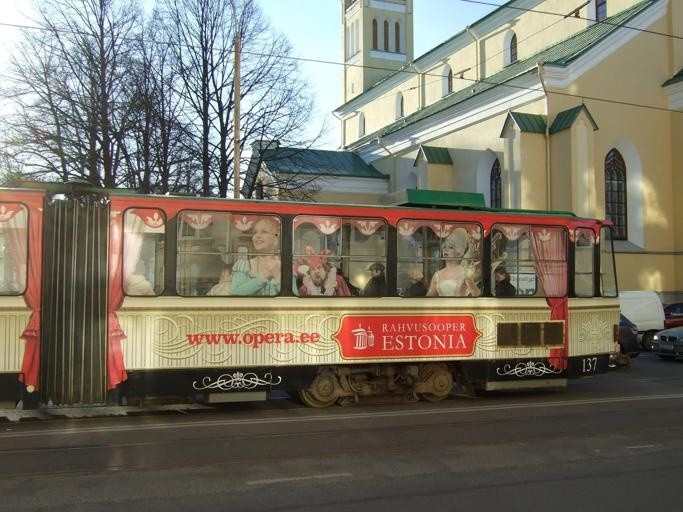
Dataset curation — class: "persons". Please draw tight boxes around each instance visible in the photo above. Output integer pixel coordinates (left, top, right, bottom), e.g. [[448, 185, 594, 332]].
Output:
[[427, 227, 481, 296], [359, 262, 384, 296], [402, 269, 426, 296], [494, 267, 515, 296], [292, 245, 350, 296], [229, 217, 298, 297]]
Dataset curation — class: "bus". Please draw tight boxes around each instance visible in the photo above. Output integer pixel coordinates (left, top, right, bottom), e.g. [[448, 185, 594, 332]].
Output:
[[0, 185, 631, 425]]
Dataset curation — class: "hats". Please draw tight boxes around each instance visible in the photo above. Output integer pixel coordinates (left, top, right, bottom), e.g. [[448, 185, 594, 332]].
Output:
[[365, 263, 385, 272]]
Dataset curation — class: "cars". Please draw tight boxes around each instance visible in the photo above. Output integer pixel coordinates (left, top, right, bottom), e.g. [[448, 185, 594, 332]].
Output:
[[617, 286, 682, 367]]
